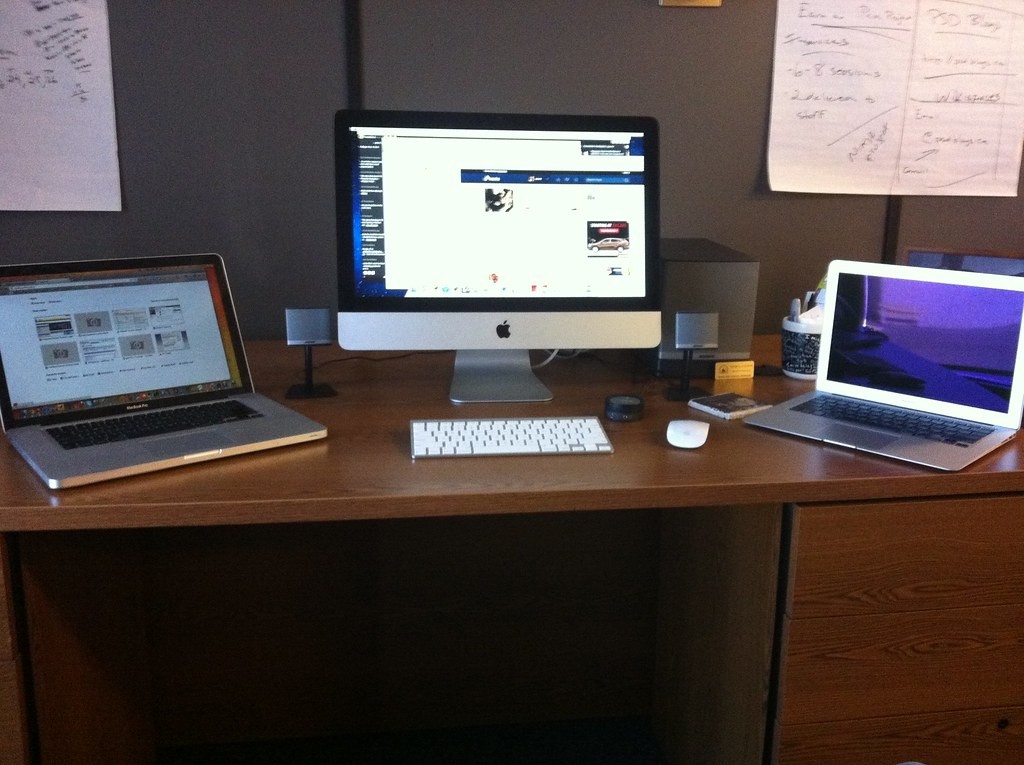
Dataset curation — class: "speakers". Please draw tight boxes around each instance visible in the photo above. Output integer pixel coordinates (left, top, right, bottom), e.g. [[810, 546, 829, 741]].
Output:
[[640, 238, 760, 377], [283, 308, 338, 398]]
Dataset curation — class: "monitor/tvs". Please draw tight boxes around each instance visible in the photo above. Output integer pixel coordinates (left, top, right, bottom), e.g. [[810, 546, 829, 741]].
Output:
[[332, 108, 663, 351]]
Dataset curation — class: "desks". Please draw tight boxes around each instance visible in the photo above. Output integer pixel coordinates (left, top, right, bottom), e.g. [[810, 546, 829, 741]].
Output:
[[0, 335, 1024, 764]]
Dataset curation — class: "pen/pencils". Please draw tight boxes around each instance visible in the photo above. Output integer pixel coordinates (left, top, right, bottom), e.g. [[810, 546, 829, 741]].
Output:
[[790, 297, 801, 323]]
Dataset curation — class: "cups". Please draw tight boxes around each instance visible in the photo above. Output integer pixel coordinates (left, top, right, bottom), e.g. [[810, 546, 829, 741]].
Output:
[[780, 316, 822, 382]]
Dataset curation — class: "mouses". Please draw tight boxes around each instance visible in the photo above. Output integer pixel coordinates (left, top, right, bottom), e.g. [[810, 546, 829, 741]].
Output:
[[666, 420, 710, 448]]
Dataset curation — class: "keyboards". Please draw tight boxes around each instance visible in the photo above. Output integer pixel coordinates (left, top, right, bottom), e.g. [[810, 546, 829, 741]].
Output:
[[409, 416, 614, 459]]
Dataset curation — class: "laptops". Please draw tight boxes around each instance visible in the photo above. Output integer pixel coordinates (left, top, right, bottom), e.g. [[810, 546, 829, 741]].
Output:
[[0, 253, 329, 490], [741, 259, 1024, 472]]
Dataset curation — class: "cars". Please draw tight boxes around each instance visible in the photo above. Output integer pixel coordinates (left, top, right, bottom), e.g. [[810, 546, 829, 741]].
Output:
[[588, 237, 630, 253]]
